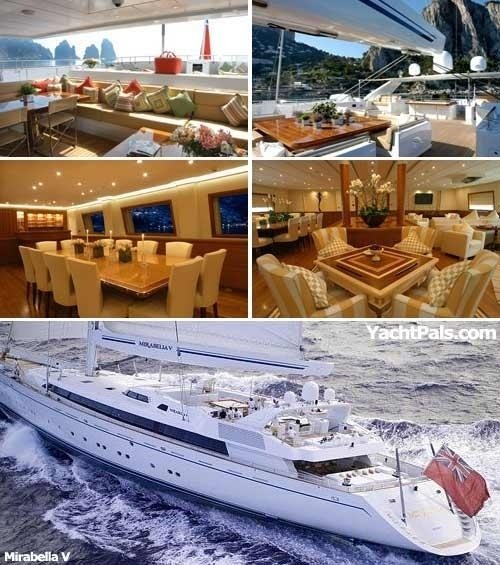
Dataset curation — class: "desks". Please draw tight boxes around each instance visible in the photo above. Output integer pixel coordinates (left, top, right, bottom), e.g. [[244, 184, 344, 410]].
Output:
[[405, 100, 457, 120], [103, 127, 193, 157]]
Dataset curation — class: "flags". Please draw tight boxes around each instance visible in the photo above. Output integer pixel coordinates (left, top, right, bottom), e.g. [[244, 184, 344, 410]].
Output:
[[423, 443, 490, 518]]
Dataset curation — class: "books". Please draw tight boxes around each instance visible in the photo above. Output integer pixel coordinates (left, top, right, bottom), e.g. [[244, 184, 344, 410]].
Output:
[[127, 140, 161, 156]]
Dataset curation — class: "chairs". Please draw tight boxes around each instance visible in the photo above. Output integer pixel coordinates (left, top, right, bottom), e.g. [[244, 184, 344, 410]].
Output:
[[0, 94, 78, 157], [252, 212, 500, 317], [252, 114, 391, 149], [18, 238, 228, 318]]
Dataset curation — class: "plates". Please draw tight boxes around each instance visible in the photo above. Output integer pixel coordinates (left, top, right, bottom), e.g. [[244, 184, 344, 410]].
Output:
[[363, 250, 374, 255]]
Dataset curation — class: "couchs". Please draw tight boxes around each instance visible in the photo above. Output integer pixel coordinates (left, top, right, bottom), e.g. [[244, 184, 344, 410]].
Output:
[[0, 80, 35, 103], [371, 113, 432, 156], [34, 79, 248, 148], [295, 135, 376, 156]]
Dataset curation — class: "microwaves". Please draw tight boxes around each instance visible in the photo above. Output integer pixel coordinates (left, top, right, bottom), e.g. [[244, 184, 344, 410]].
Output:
[[186, 61, 218, 75]]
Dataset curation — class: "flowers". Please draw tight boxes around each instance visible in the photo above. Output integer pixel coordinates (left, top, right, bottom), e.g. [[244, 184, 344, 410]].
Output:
[[346, 162, 395, 212], [169, 124, 248, 157]]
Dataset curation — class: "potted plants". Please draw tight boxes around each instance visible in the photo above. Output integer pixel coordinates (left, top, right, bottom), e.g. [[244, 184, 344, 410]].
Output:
[[81, 58, 101, 68], [16, 83, 38, 102], [295, 101, 352, 129]]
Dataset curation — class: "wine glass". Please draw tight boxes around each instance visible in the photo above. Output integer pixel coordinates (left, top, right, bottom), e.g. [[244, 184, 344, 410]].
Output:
[[370, 247, 384, 261]]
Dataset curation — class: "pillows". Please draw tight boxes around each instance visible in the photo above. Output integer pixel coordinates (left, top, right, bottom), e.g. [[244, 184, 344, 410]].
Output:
[[219, 94, 248, 127], [31, 74, 199, 118]]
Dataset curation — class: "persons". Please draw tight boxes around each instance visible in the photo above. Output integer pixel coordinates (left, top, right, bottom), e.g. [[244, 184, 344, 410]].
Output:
[[342, 473, 353, 486], [56, 363, 63, 381], [350, 434, 356, 447], [287, 427, 296, 445]]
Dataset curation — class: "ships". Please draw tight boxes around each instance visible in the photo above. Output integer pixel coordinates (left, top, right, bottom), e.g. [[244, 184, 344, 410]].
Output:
[[1, 321, 482, 557]]
